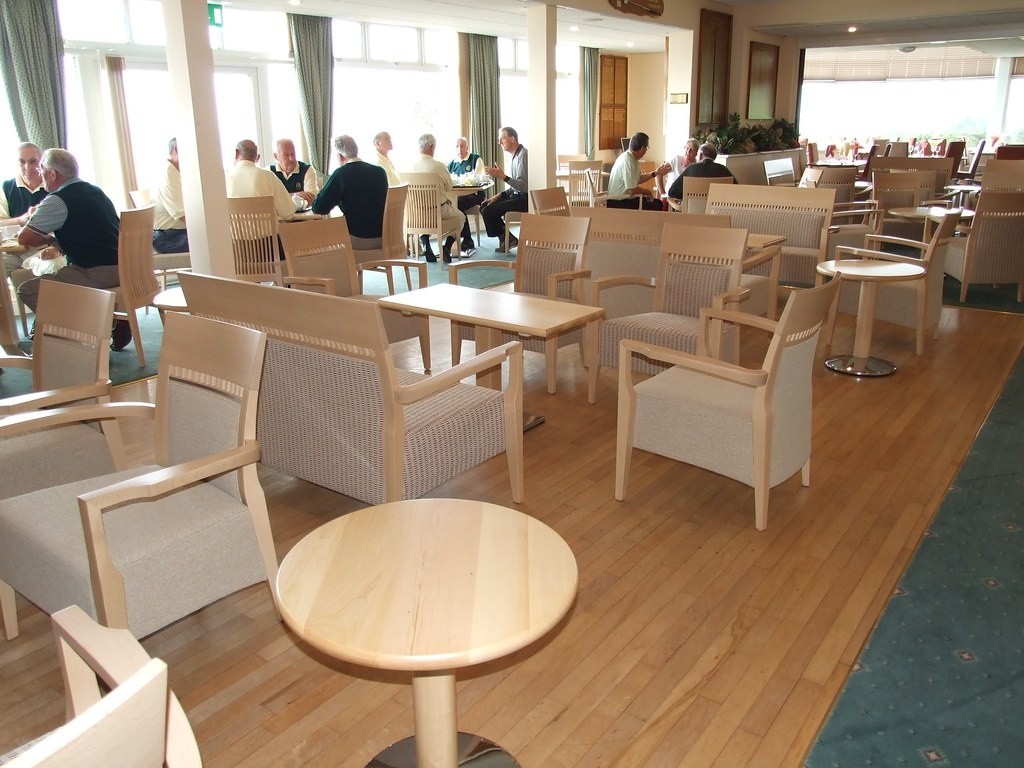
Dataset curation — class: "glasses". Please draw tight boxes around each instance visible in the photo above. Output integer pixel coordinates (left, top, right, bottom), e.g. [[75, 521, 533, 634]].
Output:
[[683, 146, 693, 153], [36, 167, 47, 177], [497, 138, 505, 141], [645, 146, 651, 150], [385, 139, 391, 144]]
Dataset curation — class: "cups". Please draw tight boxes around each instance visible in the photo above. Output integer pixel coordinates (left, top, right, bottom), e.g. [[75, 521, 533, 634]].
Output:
[[450, 173, 489, 184], [301, 200, 309, 208], [4, 225, 20, 236]]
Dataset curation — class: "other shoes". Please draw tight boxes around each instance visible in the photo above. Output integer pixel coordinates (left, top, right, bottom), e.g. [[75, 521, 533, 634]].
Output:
[[110, 318, 132, 352], [460, 240, 475, 251], [28, 319, 35, 340], [495, 237, 519, 252], [442, 245, 452, 263], [422, 243, 437, 262]]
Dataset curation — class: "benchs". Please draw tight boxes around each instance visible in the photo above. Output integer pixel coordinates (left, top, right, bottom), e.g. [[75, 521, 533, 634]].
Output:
[[704, 183, 873, 284], [177, 272, 526, 506], [570, 207, 781, 339], [868, 157, 954, 207]]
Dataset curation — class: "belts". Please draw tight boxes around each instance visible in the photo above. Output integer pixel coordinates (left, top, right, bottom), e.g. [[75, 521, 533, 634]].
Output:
[[513, 191, 518, 195], [416, 203, 446, 209], [152, 229, 164, 233]]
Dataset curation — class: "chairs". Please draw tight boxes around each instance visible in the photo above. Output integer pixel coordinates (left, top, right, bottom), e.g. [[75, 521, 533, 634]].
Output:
[[0, 134, 1024, 768]]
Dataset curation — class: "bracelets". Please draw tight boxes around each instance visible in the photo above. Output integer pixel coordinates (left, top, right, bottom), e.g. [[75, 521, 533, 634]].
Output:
[[651, 171, 657, 177]]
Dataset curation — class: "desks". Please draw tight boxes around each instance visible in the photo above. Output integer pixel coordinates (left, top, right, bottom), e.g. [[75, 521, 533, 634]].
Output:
[[377, 281, 606, 407], [943, 185, 981, 211], [434, 182, 495, 258], [817, 159, 867, 173], [275, 498, 577, 768], [556, 170, 609, 207], [816, 259, 926, 377], [887, 206, 976, 259], [277, 206, 324, 220], [747, 233, 784, 248]]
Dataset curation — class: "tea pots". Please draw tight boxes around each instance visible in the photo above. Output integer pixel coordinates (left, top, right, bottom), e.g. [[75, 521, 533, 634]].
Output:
[[289, 193, 305, 211]]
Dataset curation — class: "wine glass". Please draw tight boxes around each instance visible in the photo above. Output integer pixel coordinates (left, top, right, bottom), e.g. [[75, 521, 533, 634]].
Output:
[[852, 148, 858, 161], [909, 144, 941, 157]]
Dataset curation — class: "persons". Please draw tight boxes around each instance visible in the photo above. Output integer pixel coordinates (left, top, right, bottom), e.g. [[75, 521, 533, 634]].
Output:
[[668, 142, 739, 212], [223, 140, 297, 289], [312, 134, 389, 250], [655, 137, 701, 212], [261, 137, 320, 224], [607, 132, 667, 211], [0, 141, 49, 281], [479, 127, 528, 253], [446, 136, 485, 251], [152, 137, 190, 254], [405, 134, 466, 263], [17, 148, 132, 356], [366, 131, 426, 257]]
